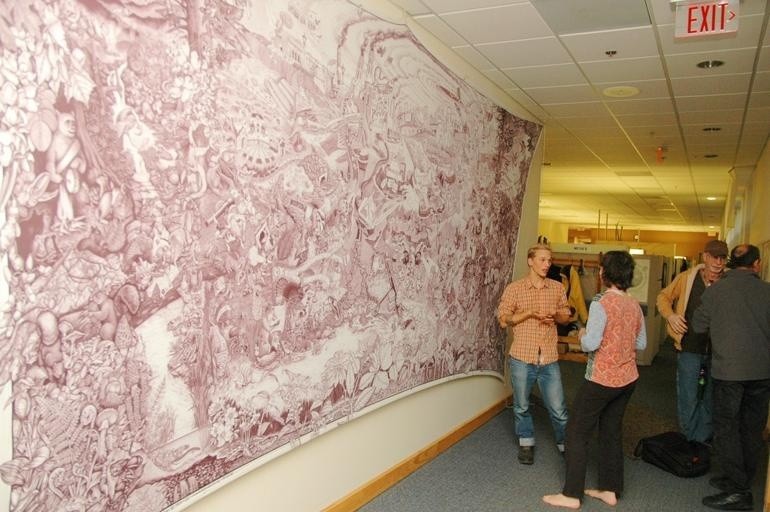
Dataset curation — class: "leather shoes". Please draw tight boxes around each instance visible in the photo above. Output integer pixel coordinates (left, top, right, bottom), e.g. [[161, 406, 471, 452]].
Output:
[[703, 478, 753, 510]]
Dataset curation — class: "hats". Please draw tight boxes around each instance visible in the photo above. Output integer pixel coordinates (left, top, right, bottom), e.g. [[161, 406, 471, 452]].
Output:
[[705, 240, 728, 257]]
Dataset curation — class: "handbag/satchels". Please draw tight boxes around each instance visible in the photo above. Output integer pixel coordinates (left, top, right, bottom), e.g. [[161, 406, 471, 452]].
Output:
[[635, 432, 708, 477]]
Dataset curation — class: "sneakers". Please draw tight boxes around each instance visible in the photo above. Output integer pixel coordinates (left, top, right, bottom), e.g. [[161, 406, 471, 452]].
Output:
[[518, 446, 534, 464]]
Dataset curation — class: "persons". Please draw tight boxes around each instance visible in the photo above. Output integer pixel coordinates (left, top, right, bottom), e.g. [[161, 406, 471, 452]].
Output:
[[495, 244, 573, 467], [688, 241, 770, 510], [655, 236, 733, 448], [542, 247, 648, 510]]
